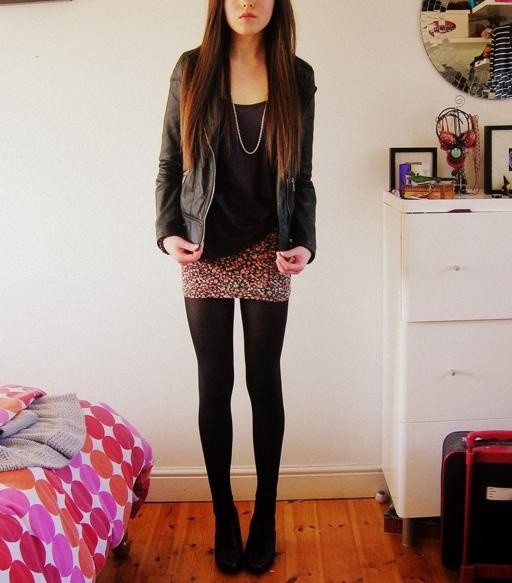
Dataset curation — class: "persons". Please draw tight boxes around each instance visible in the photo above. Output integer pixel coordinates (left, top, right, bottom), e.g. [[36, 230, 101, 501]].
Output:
[[153, 0, 320, 575]]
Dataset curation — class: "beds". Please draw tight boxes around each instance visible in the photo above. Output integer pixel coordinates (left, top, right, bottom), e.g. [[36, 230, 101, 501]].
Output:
[[0, 380, 154, 583]]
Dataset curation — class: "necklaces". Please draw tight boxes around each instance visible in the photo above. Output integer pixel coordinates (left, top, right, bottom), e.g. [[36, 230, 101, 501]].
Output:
[[230, 91, 268, 156]]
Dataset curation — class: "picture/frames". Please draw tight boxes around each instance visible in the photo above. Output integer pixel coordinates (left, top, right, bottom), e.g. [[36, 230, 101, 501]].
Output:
[[387, 146, 439, 190], [482, 123, 512, 196]]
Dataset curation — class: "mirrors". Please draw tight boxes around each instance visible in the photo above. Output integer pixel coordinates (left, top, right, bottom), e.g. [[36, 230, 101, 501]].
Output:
[[418, 0, 512, 101]]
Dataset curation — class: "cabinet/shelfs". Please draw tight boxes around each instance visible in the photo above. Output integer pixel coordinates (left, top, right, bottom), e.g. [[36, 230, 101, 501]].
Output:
[[378, 189, 512, 550]]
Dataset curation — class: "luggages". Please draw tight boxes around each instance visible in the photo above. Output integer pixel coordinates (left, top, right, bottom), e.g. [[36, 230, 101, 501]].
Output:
[[440, 429, 511, 582]]
